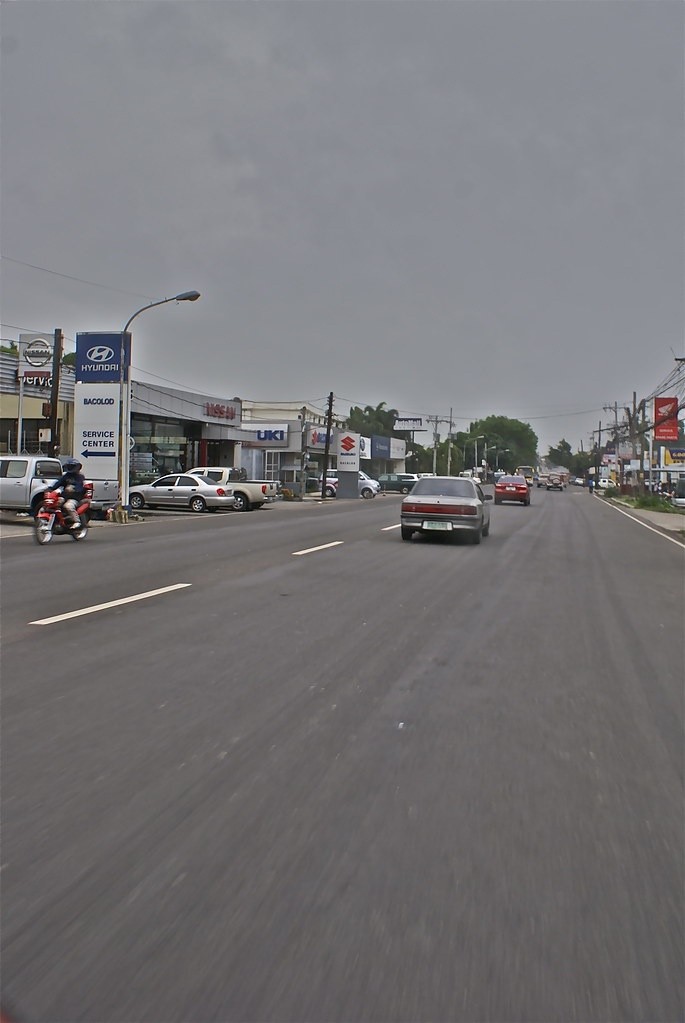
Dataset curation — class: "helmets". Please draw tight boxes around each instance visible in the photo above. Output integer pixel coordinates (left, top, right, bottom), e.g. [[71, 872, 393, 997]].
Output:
[[66, 463, 79, 473]]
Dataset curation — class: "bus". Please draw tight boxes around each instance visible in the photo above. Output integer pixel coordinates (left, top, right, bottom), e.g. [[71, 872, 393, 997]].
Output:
[[516, 466, 535, 486]]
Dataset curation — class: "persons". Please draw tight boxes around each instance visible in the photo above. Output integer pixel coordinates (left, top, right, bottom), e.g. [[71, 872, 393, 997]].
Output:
[[588, 479, 593, 493], [49, 458, 85, 529]]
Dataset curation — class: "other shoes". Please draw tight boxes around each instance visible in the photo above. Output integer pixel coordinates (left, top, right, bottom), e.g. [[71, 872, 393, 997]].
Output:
[[70, 522, 81, 530]]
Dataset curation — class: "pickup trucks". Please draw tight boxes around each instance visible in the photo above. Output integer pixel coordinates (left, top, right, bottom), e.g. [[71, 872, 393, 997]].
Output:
[[185, 467, 282, 512]]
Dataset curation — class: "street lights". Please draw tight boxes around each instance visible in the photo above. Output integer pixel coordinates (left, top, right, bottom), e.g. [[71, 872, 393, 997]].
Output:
[[107, 291, 201, 523], [496, 449, 509, 467], [463, 436, 484, 462], [484, 443, 496, 481]]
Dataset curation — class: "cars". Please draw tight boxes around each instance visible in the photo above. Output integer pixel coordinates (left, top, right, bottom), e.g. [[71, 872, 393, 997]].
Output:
[[537, 474, 549, 488], [671, 491, 685, 507], [569, 476, 595, 486], [375, 474, 418, 494], [418, 473, 436, 478], [592, 479, 616, 488], [129, 474, 235, 512], [546, 474, 564, 491], [400, 476, 493, 545], [495, 475, 533, 506]]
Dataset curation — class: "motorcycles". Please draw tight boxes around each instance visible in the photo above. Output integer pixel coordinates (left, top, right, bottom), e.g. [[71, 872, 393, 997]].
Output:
[[35, 480, 91, 545]]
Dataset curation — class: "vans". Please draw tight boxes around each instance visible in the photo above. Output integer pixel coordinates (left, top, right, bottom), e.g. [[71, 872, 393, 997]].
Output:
[[318, 469, 381, 499], [0, 456, 64, 520]]
